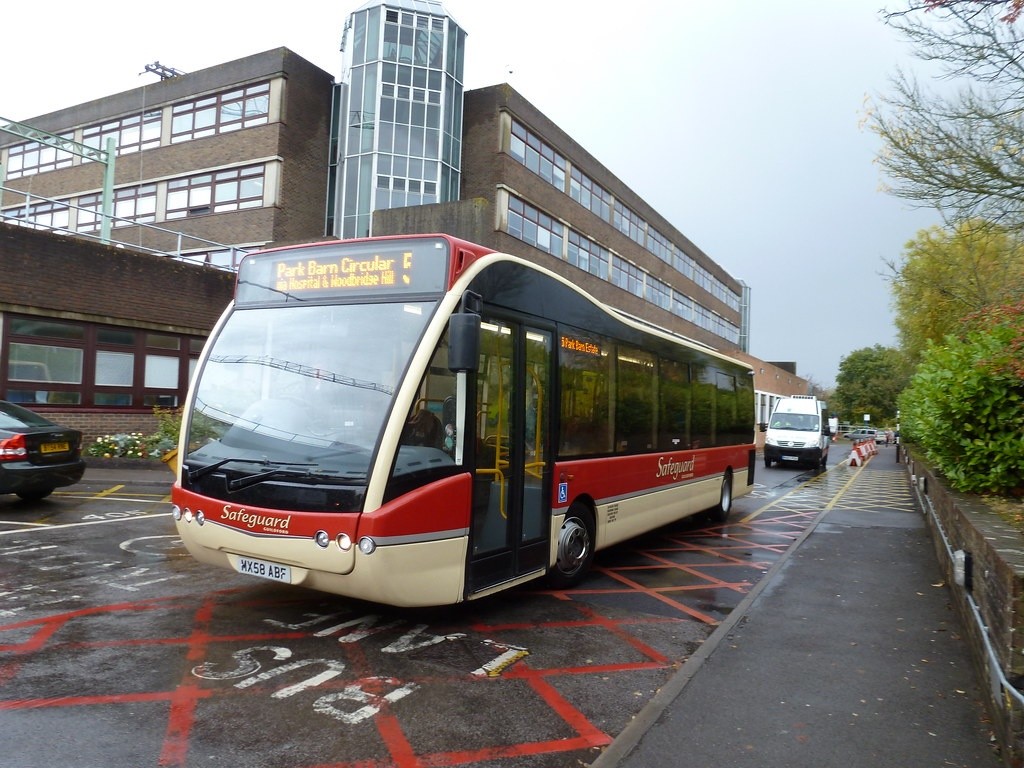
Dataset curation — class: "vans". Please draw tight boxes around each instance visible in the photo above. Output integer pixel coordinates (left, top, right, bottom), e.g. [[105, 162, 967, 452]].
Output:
[[760, 394, 830, 468]]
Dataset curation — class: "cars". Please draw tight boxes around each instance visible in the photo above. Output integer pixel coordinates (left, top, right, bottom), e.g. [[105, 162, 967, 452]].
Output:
[[0, 399, 86, 502]]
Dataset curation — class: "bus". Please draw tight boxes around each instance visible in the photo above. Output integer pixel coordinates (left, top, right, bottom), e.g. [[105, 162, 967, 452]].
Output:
[[174, 233, 759, 611]]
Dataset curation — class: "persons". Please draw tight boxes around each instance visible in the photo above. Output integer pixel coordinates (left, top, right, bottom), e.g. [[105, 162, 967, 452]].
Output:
[[888, 428, 894, 444], [773, 417, 791, 427]]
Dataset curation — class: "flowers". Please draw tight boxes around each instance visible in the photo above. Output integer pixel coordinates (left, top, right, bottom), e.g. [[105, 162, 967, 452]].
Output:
[[84, 432, 176, 460]]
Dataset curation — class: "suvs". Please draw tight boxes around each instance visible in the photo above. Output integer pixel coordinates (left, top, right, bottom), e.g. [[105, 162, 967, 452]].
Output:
[[842, 428, 887, 444]]
